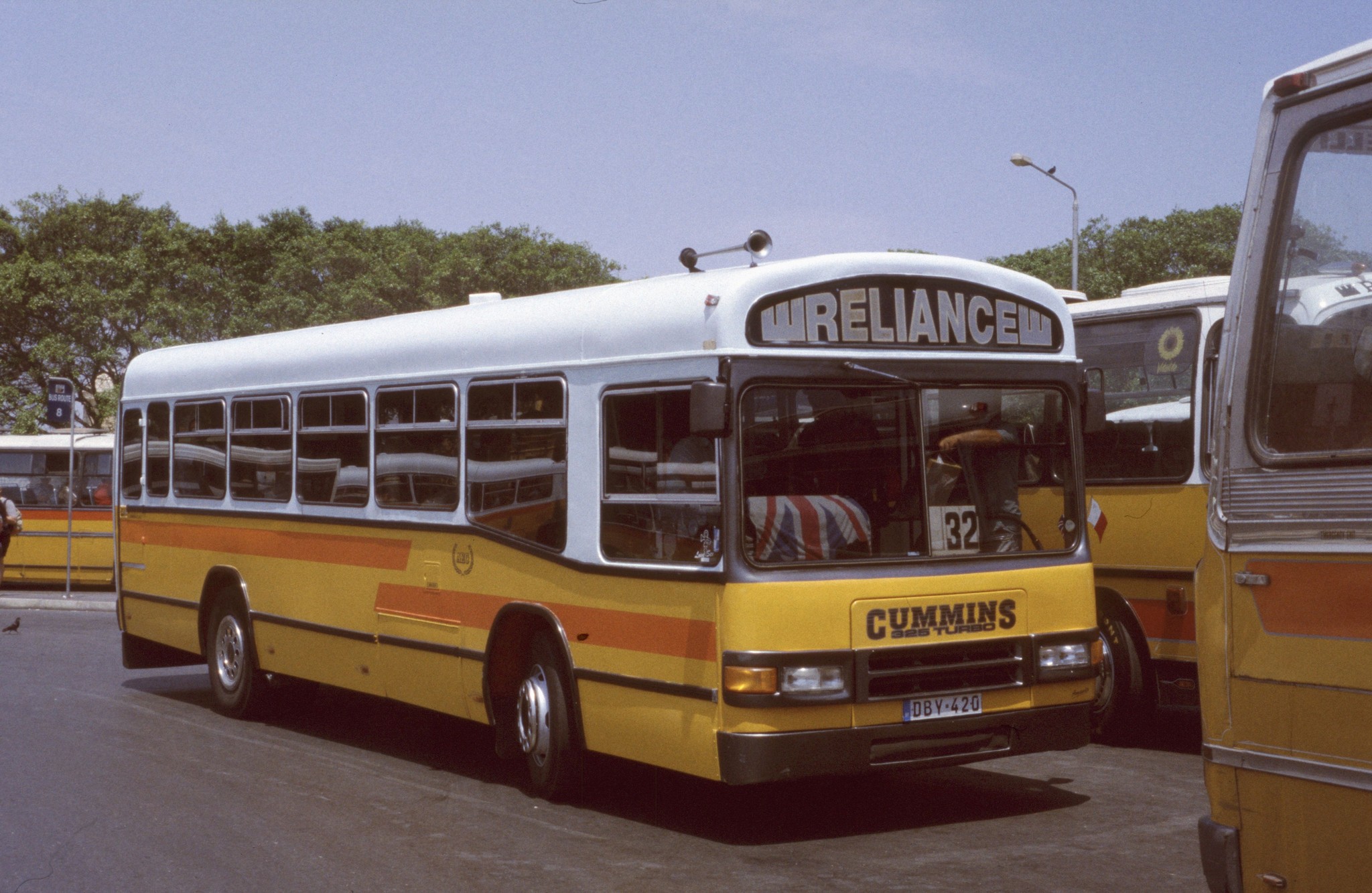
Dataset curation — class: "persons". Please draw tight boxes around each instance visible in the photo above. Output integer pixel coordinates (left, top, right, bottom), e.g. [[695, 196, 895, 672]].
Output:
[[0, 487, 17, 582], [33, 477, 112, 506]]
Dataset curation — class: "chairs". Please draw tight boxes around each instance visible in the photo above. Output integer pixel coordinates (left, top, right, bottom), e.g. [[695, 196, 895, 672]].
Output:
[[0, 482, 95, 506]]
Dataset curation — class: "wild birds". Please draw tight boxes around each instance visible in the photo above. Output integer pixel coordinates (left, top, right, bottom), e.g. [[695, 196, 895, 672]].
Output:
[[2, 617, 22, 634], [1046, 166, 1056, 176]]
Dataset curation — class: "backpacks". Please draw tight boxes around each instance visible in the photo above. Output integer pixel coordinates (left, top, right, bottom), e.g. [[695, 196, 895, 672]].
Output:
[[3, 499, 23, 537]]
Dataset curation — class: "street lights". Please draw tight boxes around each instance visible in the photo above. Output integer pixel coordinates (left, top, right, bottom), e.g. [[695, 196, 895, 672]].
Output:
[[1009, 152, 1079, 294]]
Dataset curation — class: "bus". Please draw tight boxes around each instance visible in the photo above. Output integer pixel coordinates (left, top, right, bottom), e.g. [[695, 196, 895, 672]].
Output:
[[0, 426, 115, 586], [1193, 36, 1372, 893], [115, 230, 1117, 805], [921, 263, 1372, 746]]
